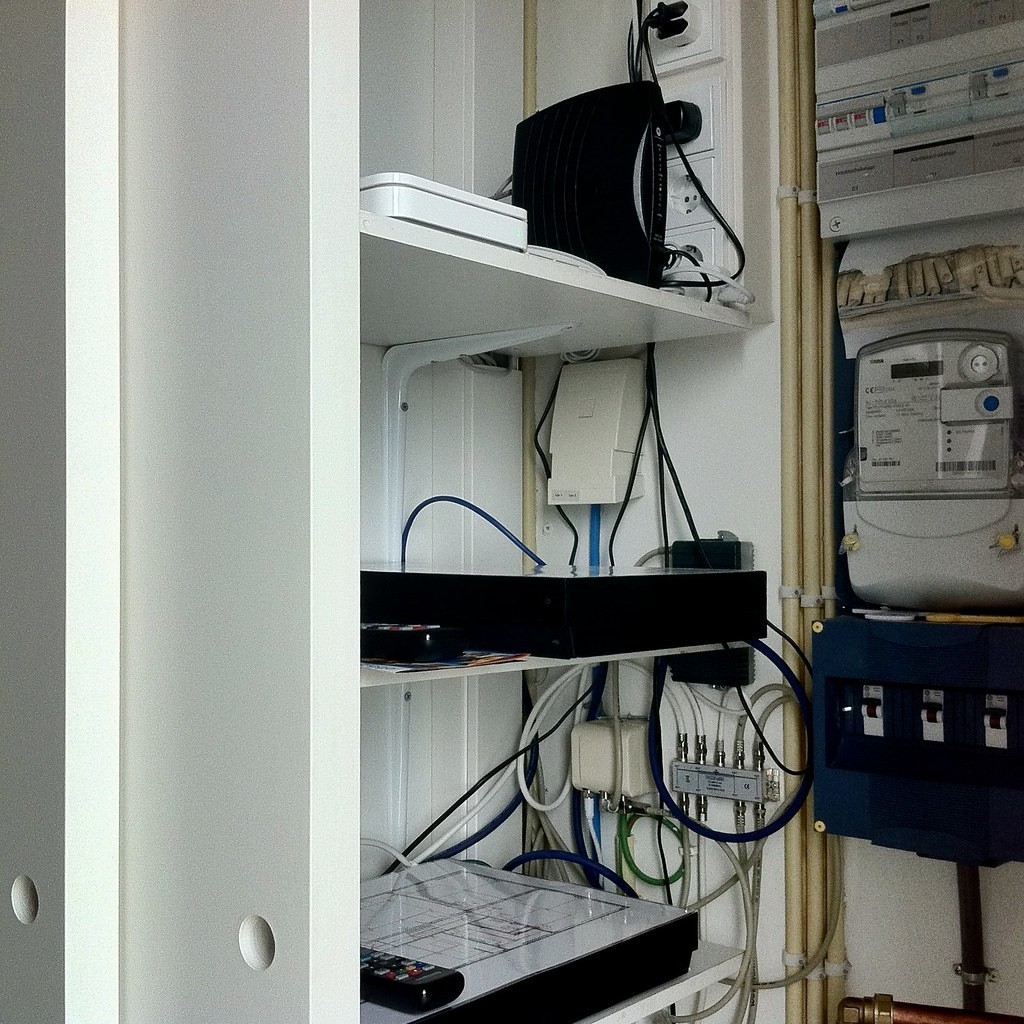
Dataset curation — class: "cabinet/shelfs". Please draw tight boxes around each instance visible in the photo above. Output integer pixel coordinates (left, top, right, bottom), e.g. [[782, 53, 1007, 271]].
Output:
[[123, 0, 780, 1024]]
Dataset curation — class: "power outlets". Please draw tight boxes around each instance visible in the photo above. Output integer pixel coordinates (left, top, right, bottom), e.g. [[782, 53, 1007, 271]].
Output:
[[662, 224, 723, 288], [662, 154, 717, 230], [649, 0, 726, 76], [658, 85, 713, 159]]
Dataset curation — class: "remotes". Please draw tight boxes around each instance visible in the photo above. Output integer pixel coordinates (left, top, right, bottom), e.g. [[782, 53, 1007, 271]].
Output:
[[360, 947, 465, 1015]]
